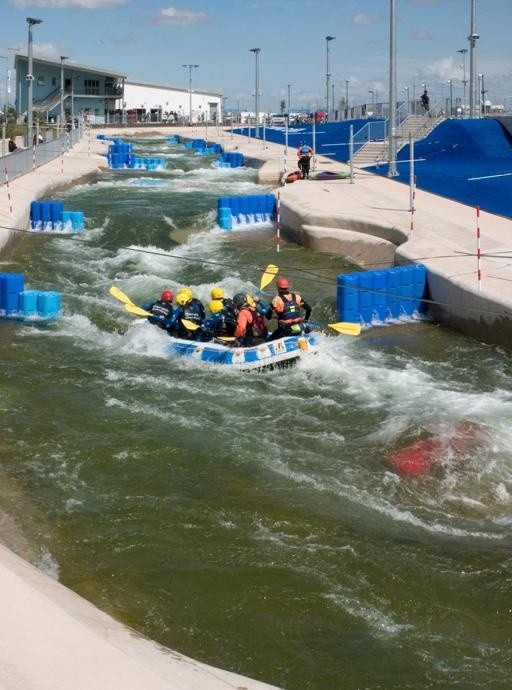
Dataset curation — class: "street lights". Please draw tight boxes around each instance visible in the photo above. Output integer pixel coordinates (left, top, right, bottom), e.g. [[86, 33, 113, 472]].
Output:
[[405, 48, 485, 118], [366, 90, 374, 104], [345, 80, 351, 109], [288, 84, 291, 123], [60, 55, 69, 137], [249, 46, 262, 137], [181, 62, 199, 124], [325, 36, 337, 122], [26, 16, 45, 148]]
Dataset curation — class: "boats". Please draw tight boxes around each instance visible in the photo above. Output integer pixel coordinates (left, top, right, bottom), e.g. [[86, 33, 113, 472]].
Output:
[[138, 301, 327, 372]]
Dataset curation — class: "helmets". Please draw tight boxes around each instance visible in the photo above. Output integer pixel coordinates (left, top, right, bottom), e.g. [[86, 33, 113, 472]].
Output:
[[233, 293, 248, 308], [179, 288, 193, 301], [276, 278, 289, 289], [161, 290, 174, 304], [208, 300, 223, 314], [210, 286, 224, 300], [175, 292, 190, 306]]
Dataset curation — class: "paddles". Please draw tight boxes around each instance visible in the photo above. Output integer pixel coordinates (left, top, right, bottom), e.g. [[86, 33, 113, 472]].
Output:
[[109, 287, 135, 306], [181, 319, 200, 331], [256, 264, 279, 295], [125, 304, 154, 316], [272, 316, 361, 336], [217, 336, 236, 341]]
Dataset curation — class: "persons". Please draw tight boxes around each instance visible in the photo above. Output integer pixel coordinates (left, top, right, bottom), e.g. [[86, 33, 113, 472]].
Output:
[[420, 89, 432, 119], [8, 135, 18, 153], [265, 277, 313, 342], [140, 110, 217, 125], [297, 139, 314, 180], [297, 140, 305, 160], [140, 287, 273, 349]]
